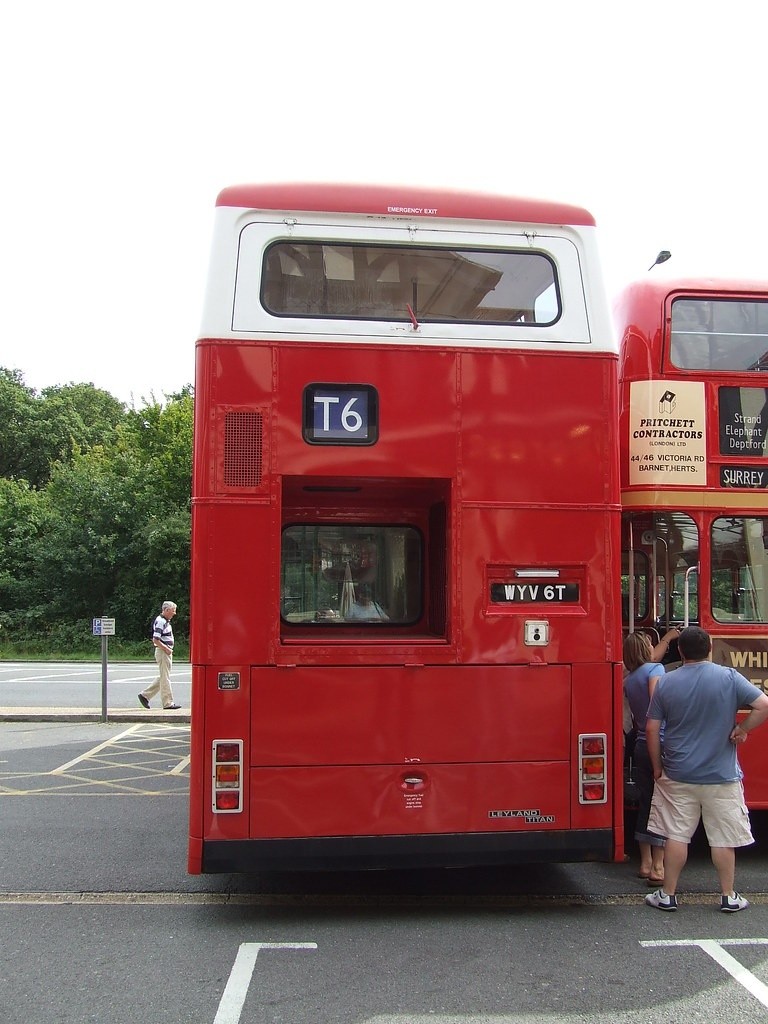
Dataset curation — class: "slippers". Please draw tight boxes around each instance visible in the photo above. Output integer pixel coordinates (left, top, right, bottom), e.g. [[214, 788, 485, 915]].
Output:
[[639, 873, 651, 878], [646, 878, 664, 887]]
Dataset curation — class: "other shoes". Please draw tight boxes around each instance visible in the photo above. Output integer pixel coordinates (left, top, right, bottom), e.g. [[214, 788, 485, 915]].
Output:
[[138, 694, 150, 709], [165, 704, 181, 709]]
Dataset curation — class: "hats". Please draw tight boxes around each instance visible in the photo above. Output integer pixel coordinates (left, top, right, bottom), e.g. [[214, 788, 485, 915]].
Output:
[[358, 586, 372, 593]]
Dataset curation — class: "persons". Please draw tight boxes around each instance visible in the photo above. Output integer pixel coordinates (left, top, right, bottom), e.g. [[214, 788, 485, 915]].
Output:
[[623, 623, 768, 913], [313, 606, 336, 621], [343, 584, 391, 623], [137, 600, 183, 709]]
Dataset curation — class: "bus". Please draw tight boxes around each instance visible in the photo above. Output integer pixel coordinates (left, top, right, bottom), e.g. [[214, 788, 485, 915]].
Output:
[[604, 275, 768, 834], [183, 177, 634, 893]]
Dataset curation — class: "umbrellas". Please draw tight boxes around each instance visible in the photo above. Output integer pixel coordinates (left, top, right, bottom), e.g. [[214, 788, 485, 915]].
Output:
[[339, 560, 357, 617]]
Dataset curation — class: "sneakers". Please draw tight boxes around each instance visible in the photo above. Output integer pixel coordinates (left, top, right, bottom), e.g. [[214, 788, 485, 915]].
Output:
[[644, 890, 678, 911], [720, 892, 750, 911]]
